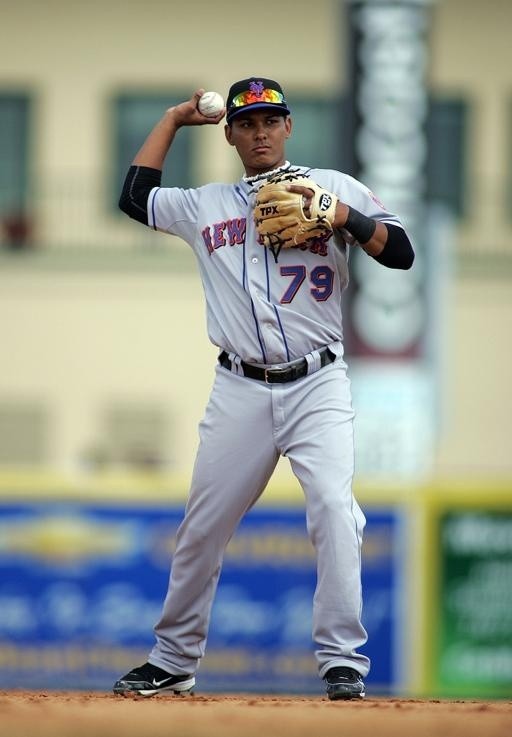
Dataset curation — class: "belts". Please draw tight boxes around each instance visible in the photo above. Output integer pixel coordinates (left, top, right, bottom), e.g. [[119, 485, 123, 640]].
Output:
[[217, 345, 336, 384]]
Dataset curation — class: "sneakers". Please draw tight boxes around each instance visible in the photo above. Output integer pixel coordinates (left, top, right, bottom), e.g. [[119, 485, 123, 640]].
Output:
[[112, 661, 196, 699], [324, 667, 366, 701]]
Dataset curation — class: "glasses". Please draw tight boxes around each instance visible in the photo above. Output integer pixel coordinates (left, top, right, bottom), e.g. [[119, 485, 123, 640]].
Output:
[[227, 89, 288, 113]]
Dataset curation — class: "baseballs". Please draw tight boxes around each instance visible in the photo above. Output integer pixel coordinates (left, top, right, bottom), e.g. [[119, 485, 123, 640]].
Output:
[[199, 91, 224, 118]]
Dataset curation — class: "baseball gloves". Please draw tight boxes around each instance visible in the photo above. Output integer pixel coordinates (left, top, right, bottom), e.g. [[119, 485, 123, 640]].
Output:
[[254, 170, 339, 264]]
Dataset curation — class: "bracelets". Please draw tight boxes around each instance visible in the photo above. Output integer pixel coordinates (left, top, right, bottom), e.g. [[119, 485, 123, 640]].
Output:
[[342, 206, 378, 244]]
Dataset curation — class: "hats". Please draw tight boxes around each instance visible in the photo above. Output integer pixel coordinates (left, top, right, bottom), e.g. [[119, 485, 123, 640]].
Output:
[[225, 77, 291, 124]]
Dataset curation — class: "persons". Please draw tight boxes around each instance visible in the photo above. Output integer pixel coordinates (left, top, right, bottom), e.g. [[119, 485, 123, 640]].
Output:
[[107, 75, 418, 702]]
